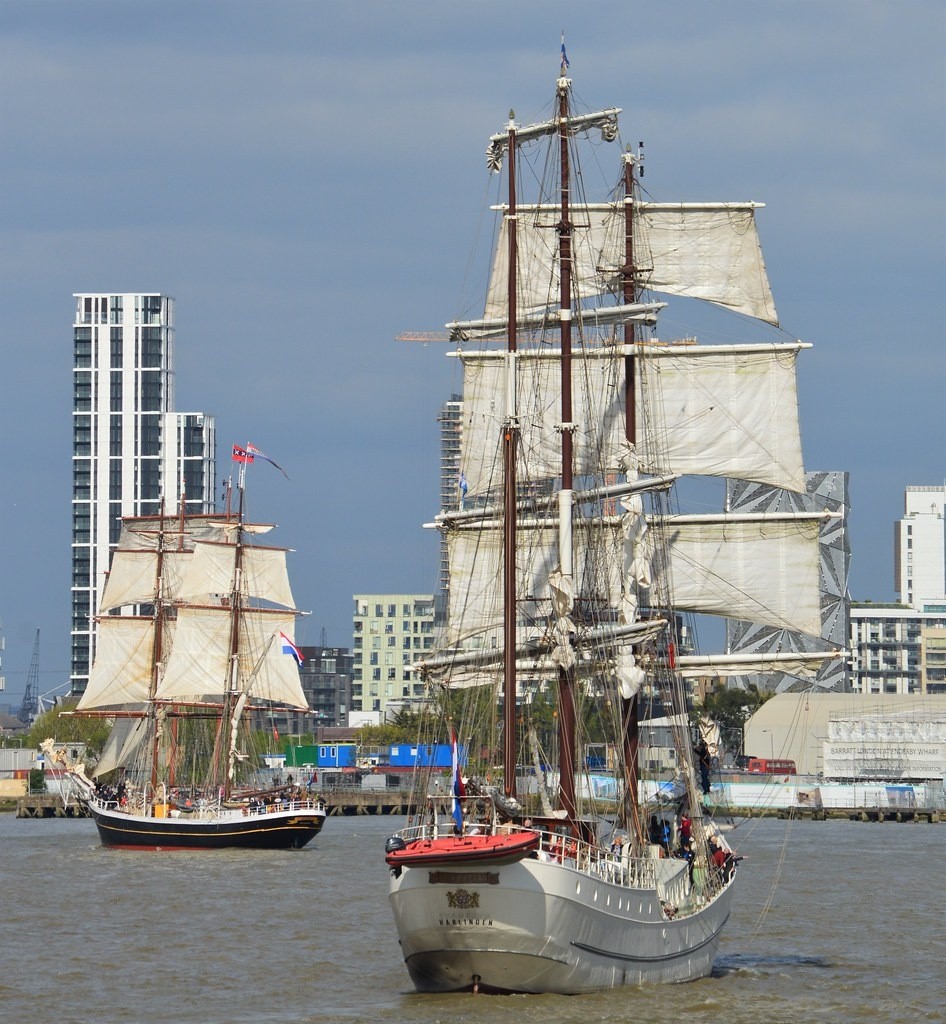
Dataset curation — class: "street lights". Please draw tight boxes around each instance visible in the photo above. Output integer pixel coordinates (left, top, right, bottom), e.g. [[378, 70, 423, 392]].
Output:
[[761, 728, 775, 775]]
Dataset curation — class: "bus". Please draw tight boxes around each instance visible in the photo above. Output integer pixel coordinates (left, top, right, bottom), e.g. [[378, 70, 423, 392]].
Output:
[[733, 754, 758, 772], [748, 758, 796, 775]]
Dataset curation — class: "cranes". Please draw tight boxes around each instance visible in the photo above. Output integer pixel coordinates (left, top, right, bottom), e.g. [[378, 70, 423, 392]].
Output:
[[16, 625, 40, 726]]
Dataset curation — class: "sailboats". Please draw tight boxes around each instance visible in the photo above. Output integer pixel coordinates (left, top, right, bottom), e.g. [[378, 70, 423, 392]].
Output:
[[381, 25, 854, 995], [55, 477, 332, 848]]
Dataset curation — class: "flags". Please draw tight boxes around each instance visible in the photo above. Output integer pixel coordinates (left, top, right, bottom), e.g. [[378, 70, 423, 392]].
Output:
[[459, 470, 469, 502], [308, 771, 318, 785], [560, 35, 570, 76], [451, 721, 467, 837], [246, 443, 289, 480], [232, 444, 255, 464], [280, 631, 305, 669]]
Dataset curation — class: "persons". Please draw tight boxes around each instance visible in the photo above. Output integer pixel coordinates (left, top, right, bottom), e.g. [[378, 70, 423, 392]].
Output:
[[462, 810, 749, 887], [92, 773, 308, 814]]
